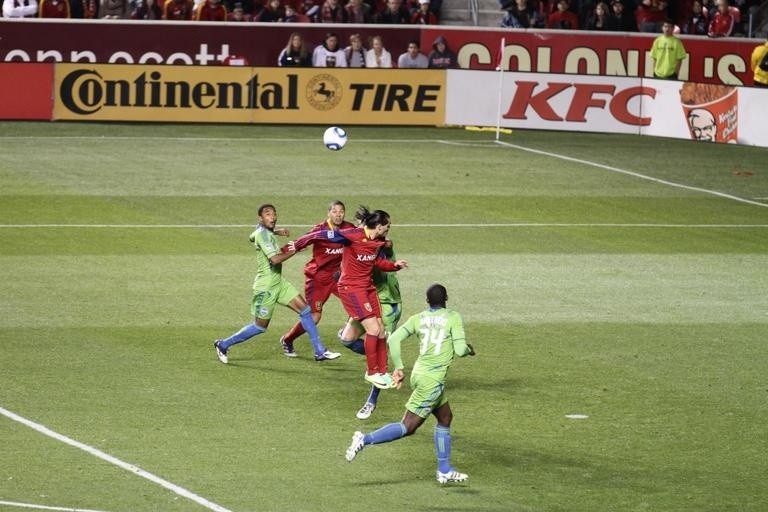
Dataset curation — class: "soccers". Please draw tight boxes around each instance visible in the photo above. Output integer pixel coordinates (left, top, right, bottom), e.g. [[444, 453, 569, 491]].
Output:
[[323, 127, 347, 150]]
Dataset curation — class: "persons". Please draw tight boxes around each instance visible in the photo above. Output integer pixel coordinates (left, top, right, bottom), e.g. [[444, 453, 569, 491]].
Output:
[[211, 203, 342, 364], [1, 0, 768, 87], [344, 283, 475, 482], [286, 206, 407, 389], [284, 199, 353, 360], [342, 238, 403, 421]]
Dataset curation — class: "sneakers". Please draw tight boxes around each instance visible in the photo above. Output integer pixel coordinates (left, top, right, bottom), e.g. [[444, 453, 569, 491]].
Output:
[[345, 430, 365, 462], [364, 369, 390, 390], [355, 400, 376, 420], [314, 350, 342, 361], [436, 470, 469, 485], [381, 372, 395, 389], [213, 339, 228, 364], [279, 335, 298, 358]]
